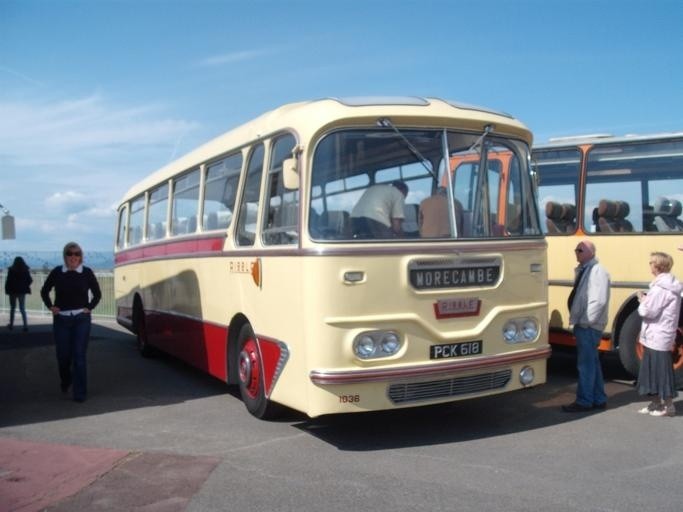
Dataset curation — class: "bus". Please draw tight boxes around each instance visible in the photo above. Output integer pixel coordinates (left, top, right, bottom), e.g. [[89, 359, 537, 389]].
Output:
[[109, 92, 561, 428], [439, 130, 683, 394]]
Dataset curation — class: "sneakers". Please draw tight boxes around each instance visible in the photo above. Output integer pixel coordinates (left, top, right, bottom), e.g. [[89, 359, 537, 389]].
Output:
[[6, 323, 30, 332], [60, 378, 88, 404], [561, 400, 608, 413]]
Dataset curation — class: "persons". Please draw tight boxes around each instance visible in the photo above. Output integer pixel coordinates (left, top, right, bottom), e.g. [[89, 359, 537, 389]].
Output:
[[561, 241, 610, 413], [348, 180, 409, 237], [417, 186, 462, 238], [636, 252, 683, 416], [41, 242, 101, 403], [5, 257, 33, 333]]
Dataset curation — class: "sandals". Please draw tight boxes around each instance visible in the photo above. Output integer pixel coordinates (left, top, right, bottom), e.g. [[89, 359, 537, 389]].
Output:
[[637, 403, 677, 418]]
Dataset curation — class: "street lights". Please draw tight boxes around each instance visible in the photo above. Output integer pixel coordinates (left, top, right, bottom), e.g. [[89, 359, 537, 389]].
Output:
[[0, 204, 17, 241]]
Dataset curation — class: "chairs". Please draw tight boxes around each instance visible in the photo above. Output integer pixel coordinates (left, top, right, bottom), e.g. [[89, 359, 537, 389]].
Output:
[[546, 200, 683, 235], [138, 199, 420, 243]]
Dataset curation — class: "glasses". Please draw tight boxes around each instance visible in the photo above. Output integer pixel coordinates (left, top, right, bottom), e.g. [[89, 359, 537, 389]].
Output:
[[574, 248, 590, 253], [65, 250, 81, 257], [647, 260, 658, 266]]
